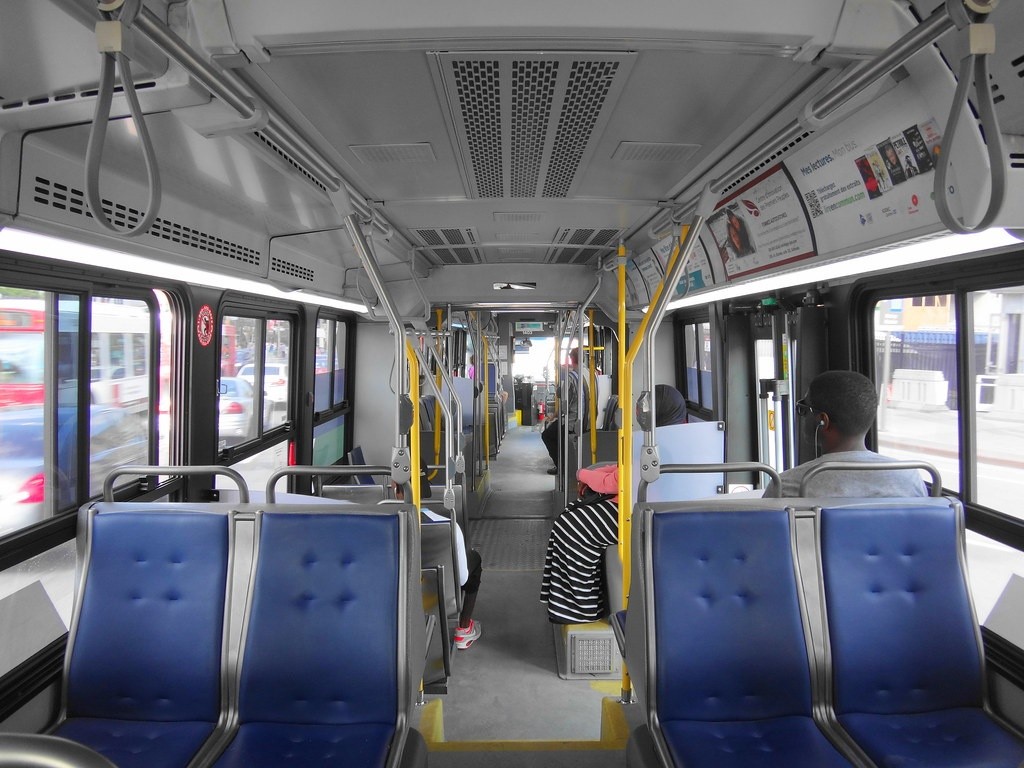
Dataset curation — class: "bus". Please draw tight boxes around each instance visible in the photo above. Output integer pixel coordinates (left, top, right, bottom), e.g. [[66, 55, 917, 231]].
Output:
[[1, 298, 152, 458], [219, 321, 237, 379]]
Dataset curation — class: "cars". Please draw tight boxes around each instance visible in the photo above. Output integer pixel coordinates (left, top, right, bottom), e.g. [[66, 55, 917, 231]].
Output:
[[0, 402, 171, 570], [162, 376, 273, 446], [230, 362, 292, 413]]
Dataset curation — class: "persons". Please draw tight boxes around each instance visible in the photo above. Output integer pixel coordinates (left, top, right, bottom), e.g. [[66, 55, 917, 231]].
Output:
[[392, 457, 483, 649], [539, 384, 689, 626], [541, 347, 597, 474], [724, 209, 757, 260], [761, 370, 928, 499], [469, 354, 509, 404], [885, 145, 896, 165]]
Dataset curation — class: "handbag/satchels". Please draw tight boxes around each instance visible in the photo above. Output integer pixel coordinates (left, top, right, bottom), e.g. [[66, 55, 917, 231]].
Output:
[[577, 484, 617, 506]]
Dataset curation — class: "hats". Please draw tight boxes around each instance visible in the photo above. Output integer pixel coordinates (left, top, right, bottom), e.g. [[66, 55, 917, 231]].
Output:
[[420, 459, 432, 499]]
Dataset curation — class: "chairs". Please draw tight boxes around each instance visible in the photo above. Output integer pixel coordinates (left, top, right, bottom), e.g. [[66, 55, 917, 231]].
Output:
[[0, 360, 506, 768], [567, 393, 1024, 768]]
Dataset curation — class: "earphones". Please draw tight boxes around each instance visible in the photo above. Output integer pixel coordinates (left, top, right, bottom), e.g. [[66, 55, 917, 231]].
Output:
[[819, 420, 825, 427]]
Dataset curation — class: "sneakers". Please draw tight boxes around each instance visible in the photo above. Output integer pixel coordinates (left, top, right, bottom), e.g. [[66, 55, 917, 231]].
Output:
[[452, 620, 483, 649]]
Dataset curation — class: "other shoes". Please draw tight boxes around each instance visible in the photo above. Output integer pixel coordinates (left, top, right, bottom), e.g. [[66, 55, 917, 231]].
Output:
[[547, 466, 558, 475]]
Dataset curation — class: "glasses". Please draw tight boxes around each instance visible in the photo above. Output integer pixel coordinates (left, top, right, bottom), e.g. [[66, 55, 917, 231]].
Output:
[[795, 398, 823, 417]]
